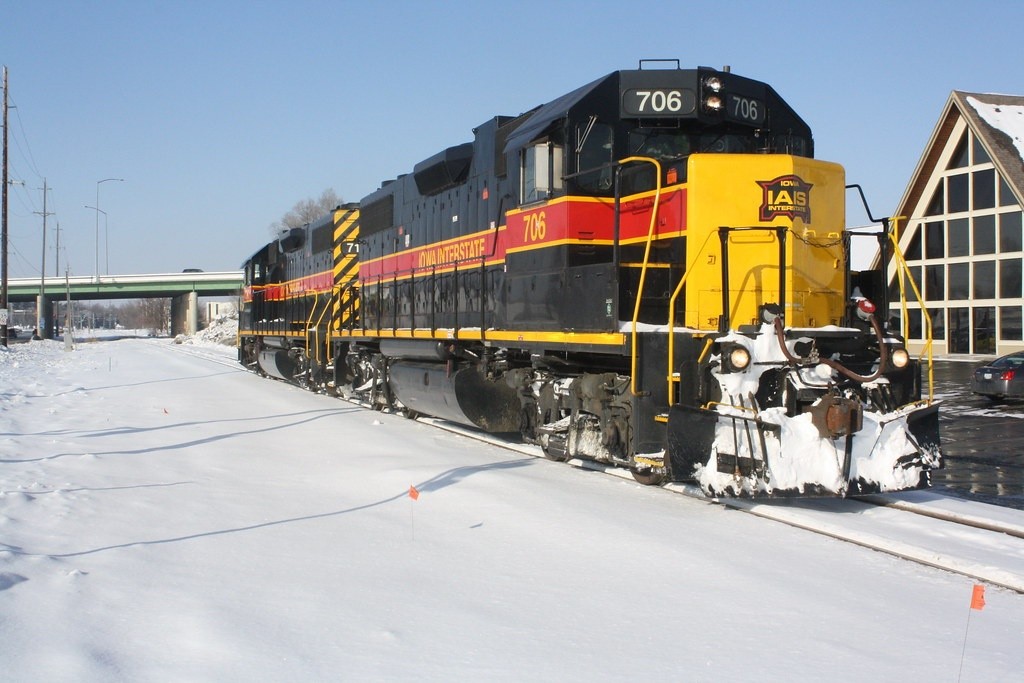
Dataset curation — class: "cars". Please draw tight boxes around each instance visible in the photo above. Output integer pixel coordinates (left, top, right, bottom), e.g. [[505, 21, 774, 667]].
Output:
[[183, 269, 204, 272], [970, 349, 1024, 407]]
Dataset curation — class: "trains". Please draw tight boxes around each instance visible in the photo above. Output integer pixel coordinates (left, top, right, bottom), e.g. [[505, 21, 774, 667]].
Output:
[[231, 55, 945, 509]]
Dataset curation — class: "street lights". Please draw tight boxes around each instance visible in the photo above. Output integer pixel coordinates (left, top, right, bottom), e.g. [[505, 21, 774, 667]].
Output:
[[95, 178, 123, 294], [85, 206, 109, 276]]
